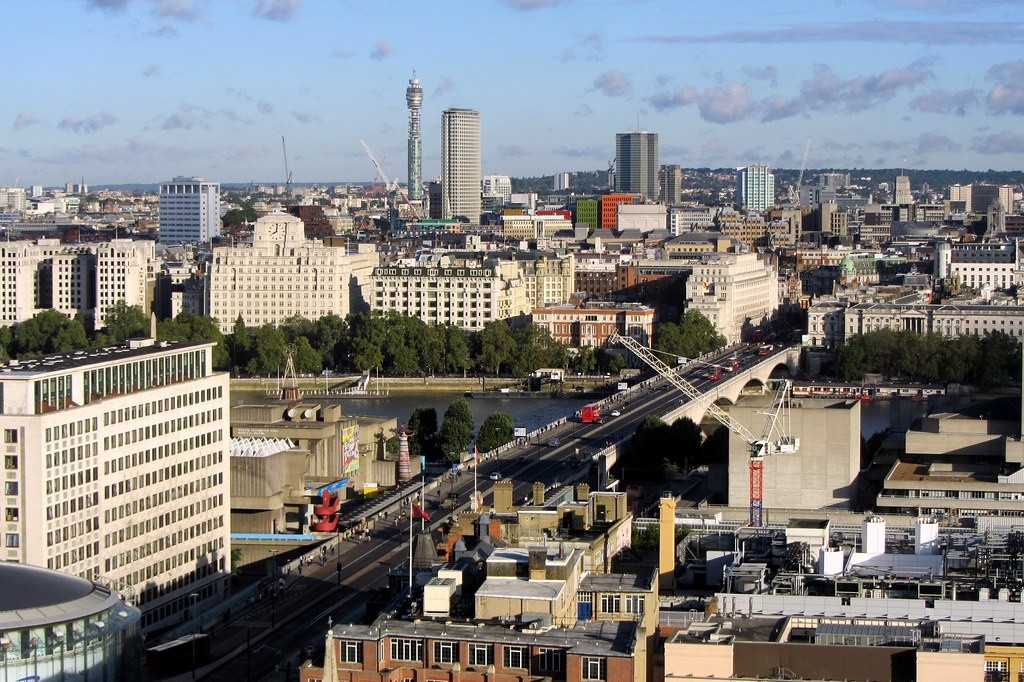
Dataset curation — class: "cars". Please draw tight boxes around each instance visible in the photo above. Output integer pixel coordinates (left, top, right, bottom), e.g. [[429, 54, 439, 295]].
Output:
[[596, 418, 605, 424], [611, 410, 620, 417], [518, 456, 528, 463], [490, 472, 502, 481], [548, 438, 560, 448], [769, 345, 774, 352], [778, 342, 784, 348]]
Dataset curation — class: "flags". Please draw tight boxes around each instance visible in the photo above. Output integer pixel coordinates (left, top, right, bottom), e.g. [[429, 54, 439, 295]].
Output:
[[412, 504, 429, 519]]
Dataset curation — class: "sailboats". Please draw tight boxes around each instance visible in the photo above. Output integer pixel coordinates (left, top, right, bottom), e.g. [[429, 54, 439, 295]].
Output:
[[264, 352, 390, 399]]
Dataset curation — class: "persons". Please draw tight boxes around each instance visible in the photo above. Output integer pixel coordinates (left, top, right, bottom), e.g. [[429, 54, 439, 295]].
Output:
[[605, 381, 652, 407], [655, 340, 737, 382], [344, 524, 374, 544], [317, 545, 337, 566], [496, 417, 568, 458], [401, 490, 443, 506], [299, 555, 316, 570], [376, 507, 405, 528]]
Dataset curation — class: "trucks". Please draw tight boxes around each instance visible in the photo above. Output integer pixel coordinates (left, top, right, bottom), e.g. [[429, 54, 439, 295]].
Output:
[[439, 493, 461, 510]]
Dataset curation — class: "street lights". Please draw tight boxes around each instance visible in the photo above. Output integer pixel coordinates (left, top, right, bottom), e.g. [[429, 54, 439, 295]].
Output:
[[189, 592, 199, 682], [269, 549, 278, 629], [495, 428, 501, 472], [398, 479, 404, 547], [335, 512, 343, 587]]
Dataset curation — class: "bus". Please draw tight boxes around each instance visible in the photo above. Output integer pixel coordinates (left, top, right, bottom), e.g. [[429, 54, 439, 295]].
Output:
[[708, 364, 721, 381], [726, 357, 739, 372], [582, 403, 600, 423], [759, 345, 770, 356]]
